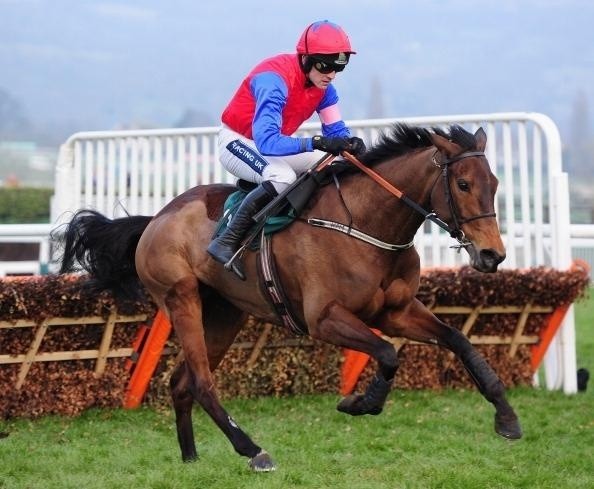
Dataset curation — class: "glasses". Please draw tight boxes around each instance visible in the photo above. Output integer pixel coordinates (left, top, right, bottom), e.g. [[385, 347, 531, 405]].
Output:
[[305, 55, 346, 74]]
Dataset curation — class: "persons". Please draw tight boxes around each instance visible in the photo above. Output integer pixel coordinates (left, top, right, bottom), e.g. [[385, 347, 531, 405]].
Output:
[[204, 18, 366, 281]]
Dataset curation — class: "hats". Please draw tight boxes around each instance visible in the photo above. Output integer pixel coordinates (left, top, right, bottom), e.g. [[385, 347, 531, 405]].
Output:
[[296, 19, 356, 57]]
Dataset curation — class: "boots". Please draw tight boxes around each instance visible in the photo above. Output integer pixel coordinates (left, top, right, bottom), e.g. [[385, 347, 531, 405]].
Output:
[[205, 183, 278, 281]]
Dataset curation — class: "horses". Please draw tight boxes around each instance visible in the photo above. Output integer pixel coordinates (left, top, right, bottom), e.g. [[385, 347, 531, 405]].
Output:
[[51, 120, 523, 475]]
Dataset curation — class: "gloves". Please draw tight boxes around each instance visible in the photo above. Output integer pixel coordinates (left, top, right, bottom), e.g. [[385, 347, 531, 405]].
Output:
[[345, 135, 369, 159], [313, 133, 349, 157]]
